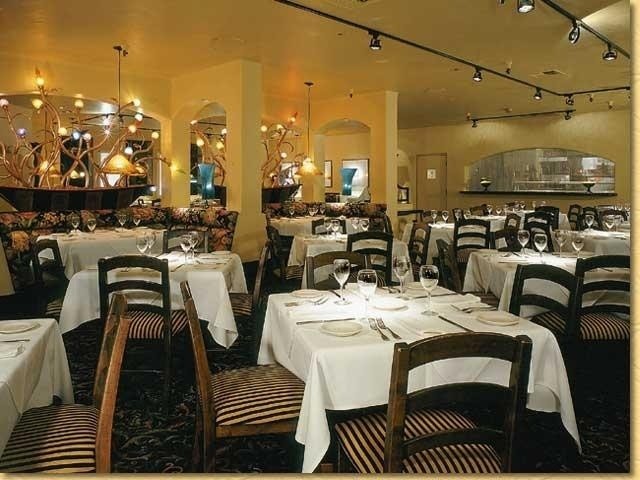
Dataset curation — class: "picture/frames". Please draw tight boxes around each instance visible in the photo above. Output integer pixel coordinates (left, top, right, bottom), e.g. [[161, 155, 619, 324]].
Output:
[[325, 160, 332, 187]]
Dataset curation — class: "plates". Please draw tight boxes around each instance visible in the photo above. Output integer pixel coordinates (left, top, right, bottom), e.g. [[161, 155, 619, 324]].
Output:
[[476, 311, 520, 326], [0, 320, 39, 334], [371, 296, 407, 311], [319, 321, 364, 338], [406, 281, 426, 290], [290, 287, 322, 298]]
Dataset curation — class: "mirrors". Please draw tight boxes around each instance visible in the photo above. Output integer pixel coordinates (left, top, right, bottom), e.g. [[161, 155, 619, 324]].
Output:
[[342, 158, 370, 202]]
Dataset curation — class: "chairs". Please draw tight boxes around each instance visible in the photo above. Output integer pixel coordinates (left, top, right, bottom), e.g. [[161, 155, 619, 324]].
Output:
[[1, 291, 134, 475], [179, 279, 306, 474], [2, 205, 631, 408], [335, 331, 534, 474]]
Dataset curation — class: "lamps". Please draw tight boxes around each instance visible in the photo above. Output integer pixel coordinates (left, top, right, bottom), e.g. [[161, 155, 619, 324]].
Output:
[[116, 49, 125, 126], [0, 77, 311, 188], [470, 0, 619, 127], [367, 35, 382, 51]]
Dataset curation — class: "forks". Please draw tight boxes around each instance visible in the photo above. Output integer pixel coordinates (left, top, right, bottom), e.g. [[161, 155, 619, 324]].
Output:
[[284, 295, 331, 309], [366, 315, 401, 341]]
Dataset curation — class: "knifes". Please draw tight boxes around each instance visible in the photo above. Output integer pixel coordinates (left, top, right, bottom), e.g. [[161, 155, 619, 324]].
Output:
[[415, 293, 458, 299], [328, 288, 347, 301], [295, 316, 357, 326], [438, 315, 474, 331]]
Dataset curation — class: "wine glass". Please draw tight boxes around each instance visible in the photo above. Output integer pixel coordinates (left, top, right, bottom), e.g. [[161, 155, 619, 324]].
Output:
[[332, 258, 352, 306], [322, 215, 369, 241], [480, 183, 492, 192], [288, 206, 295, 218], [516, 228, 584, 259], [585, 200, 630, 235], [71, 212, 141, 240], [418, 264, 439, 317], [135, 230, 200, 267], [393, 255, 412, 301], [357, 268, 377, 323], [429, 199, 546, 225], [583, 182, 595, 194], [308, 205, 327, 217]]
